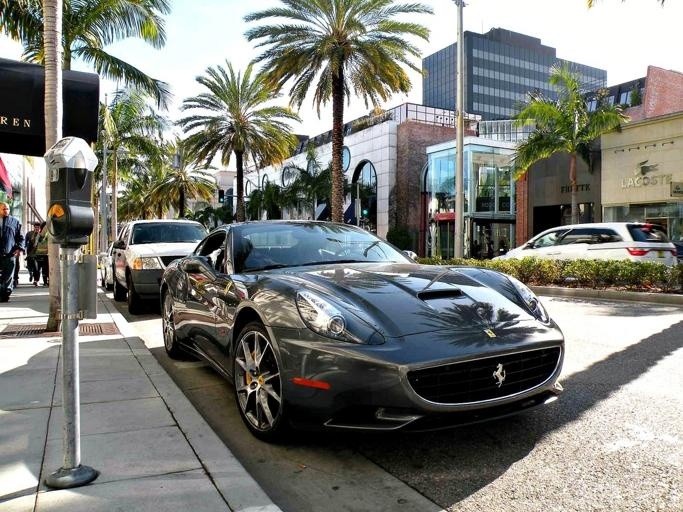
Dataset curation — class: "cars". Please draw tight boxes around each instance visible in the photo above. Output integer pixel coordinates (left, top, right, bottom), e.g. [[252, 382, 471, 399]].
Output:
[[491, 221, 678, 267], [100, 219, 208, 316]]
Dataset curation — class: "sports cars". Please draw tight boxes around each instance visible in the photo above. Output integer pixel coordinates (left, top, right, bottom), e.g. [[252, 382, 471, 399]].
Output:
[[160, 220, 565, 441]]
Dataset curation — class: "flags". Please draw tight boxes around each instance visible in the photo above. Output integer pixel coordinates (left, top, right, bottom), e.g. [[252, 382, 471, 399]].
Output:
[[0, 156, 15, 200]]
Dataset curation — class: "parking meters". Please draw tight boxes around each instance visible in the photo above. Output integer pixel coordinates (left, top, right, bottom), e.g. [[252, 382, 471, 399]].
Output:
[[44, 135, 99, 470]]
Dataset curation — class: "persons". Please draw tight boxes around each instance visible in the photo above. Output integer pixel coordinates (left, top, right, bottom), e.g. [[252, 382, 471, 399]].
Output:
[[33, 220, 49, 287], [24, 222, 43, 282], [488, 239, 495, 258], [0, 201, 25, 302], [497, 240, 508, 255]]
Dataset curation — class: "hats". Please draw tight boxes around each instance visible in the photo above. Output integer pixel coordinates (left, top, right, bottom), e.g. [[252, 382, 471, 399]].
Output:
[[39, 222, 46, 233]]
